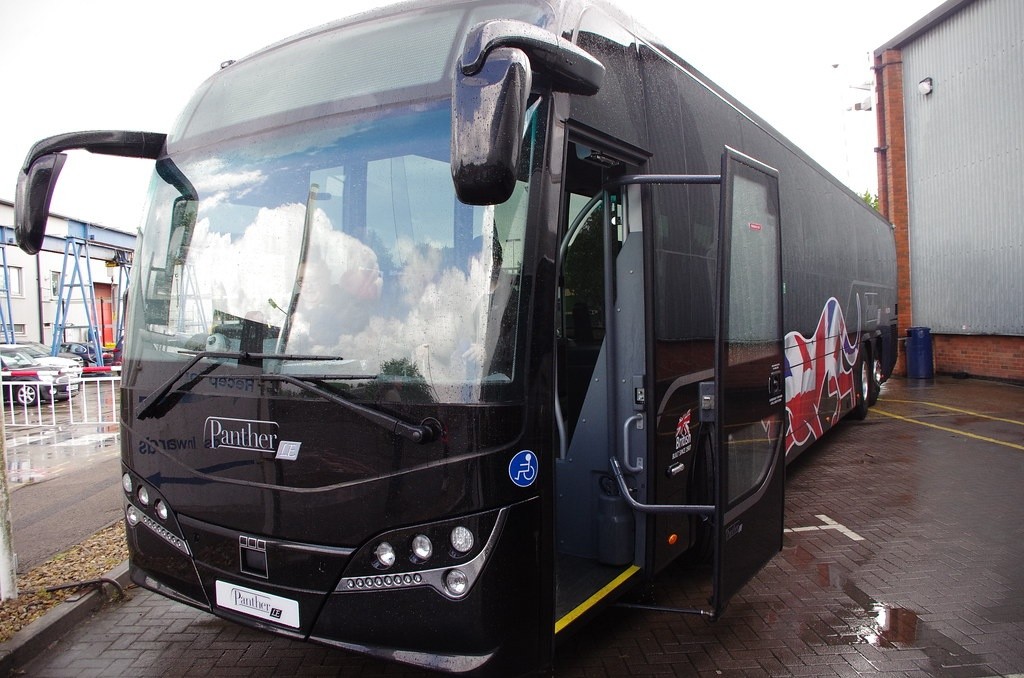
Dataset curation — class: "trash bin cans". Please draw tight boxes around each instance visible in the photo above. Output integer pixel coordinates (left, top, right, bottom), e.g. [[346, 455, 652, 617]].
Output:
[[903, 326, 935, 380]]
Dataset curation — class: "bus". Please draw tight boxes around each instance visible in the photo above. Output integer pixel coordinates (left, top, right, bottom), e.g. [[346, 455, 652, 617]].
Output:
[[7, 0, 903, 678]]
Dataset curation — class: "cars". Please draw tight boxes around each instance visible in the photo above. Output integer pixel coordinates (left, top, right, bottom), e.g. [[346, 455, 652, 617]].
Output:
[[59, 341, 115, 378], [1, 343, 84, 378], [39, 345, 84, 368], [0, 347, 79, 405]]
